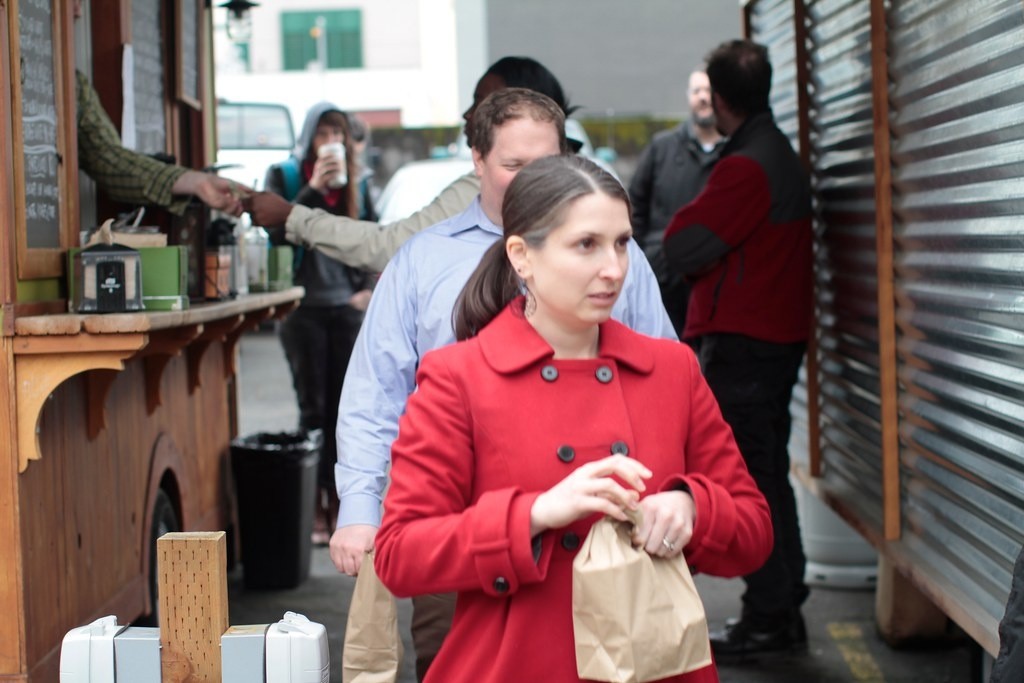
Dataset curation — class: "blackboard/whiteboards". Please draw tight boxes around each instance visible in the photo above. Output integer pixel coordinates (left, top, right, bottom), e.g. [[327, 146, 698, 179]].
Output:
[[127, 0, 171, 156], [171, 0, 203, 111], [8, 0, 70, 283]]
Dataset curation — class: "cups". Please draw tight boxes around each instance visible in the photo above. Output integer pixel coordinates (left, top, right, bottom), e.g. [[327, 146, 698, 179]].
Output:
[[317, 142, 347, 189]]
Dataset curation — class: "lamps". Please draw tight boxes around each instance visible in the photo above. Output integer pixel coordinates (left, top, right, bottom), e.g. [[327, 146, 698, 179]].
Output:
[[216, 0, 261, 58]]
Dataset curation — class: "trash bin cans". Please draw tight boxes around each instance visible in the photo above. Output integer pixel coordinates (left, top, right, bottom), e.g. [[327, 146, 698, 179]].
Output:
[[231, 429, 322, 592]]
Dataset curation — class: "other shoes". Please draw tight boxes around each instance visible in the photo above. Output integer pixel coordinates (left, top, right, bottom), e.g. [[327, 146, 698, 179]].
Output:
[[711, 609, 809, 665], [308, 508, 331, 546]]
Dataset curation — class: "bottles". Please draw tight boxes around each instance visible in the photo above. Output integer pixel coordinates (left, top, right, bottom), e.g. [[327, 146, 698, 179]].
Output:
[[245, 226, 269, 291], [205, 219, 235, 298]]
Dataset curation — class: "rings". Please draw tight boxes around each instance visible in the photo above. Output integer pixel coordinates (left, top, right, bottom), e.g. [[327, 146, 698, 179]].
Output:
[[663, 537, 675, 552]]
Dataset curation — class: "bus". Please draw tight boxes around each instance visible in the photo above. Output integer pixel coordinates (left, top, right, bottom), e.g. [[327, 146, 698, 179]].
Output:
[[212, 96, 301, 197]]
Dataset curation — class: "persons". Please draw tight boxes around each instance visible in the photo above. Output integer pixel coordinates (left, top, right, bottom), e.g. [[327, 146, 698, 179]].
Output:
[[375, 155, 774, 682], [328, 87, 680, 683], [664, 40, 815, 658], [239, 56, 566, 683], [73, 0, 254, 217], [263, 101, 379, 544], [628, 71, 729, 345]]
